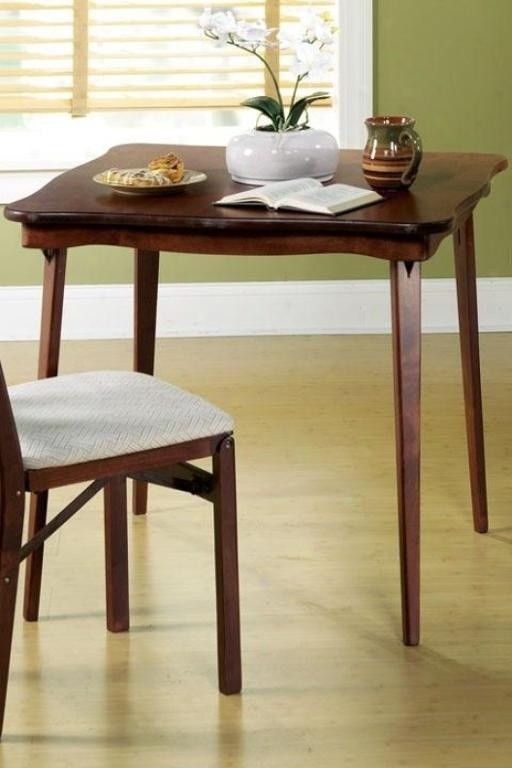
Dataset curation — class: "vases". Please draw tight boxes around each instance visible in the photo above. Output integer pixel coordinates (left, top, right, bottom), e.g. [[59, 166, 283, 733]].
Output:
[[225, 104, 338, 187]]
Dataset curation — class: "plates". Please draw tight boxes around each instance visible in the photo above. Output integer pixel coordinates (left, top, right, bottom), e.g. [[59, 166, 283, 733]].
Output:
[[91, 166, 208, 196]]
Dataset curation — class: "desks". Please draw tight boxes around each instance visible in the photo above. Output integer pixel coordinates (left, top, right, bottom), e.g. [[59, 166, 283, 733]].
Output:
[[4, 142, 508, 643]]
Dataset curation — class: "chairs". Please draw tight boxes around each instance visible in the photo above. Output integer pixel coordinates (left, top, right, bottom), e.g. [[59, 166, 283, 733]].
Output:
[[1, 359, 241, 737]]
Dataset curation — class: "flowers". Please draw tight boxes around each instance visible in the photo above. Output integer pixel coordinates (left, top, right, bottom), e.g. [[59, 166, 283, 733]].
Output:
[[187, 2, 342, 133]]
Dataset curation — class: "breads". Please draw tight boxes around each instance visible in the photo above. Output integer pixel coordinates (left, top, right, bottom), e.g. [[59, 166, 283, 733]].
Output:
[[101, 153, 185, 189]]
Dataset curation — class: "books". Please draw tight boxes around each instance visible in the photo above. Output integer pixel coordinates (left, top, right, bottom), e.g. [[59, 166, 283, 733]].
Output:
[[212, 173, 384, 216]]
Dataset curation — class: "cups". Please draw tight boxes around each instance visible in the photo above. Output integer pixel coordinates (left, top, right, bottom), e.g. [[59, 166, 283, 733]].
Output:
[[361, 115, 424, 195]]
